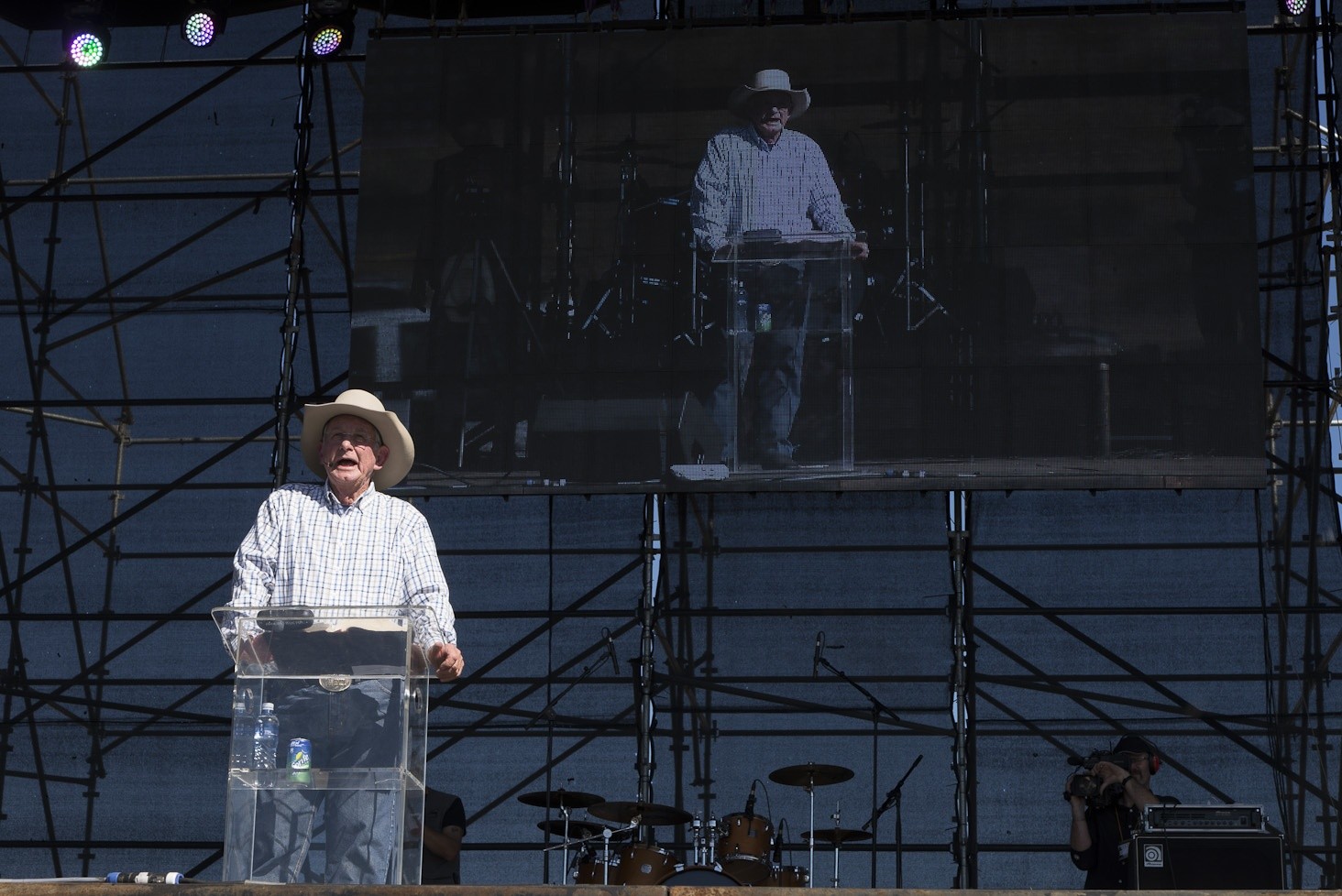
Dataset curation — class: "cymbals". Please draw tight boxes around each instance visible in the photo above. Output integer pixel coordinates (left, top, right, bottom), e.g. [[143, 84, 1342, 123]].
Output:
[[859, 117, 953, 129], [517, 791, 606, 809], [586, 801, 694, 827], [536, 819, 632, 842], [800, 828, 875, 842], [768, 763, 855, 787]]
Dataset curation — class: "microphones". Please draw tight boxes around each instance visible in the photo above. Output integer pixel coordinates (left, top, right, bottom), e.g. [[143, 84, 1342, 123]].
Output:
[[606, 628, 620, 675], [323, 461, 333, 467], [1068, 755, 1084, 766], [745, 781, 757, 812], [813, 633, 821, 680], [772, 821, 783, 862]]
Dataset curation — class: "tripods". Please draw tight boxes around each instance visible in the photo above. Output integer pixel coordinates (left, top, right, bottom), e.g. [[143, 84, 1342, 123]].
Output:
[[579, 157, 638, 341], [867, 102, 965, 333], [435, 185, 560, 469]]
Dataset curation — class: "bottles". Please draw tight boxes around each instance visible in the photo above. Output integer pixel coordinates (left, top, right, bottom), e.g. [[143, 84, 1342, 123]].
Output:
[[231, 703, 253, 769], [734, 281, 748, 333], [253, 702, 280, 770]]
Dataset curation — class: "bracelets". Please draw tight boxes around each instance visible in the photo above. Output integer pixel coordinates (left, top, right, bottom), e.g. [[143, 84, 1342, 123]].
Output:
[[1073, 818, 1086, 822], [1121, 775, 1133, 786]]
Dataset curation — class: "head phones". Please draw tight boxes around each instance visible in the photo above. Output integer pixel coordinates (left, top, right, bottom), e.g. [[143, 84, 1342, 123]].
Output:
[[1120, 733, 1163, 775]]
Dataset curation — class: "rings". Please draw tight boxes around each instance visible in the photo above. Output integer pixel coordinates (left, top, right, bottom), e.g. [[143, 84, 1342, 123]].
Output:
[[867, 250, 869, 254]]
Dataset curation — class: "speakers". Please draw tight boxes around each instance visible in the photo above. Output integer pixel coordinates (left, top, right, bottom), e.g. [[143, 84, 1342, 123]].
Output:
[[1116, 834, 1287, 890], [533, 390, 726, 483]]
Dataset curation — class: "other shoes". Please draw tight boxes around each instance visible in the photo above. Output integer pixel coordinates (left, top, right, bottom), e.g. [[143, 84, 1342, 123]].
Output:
[[762, 455, 797, 470]]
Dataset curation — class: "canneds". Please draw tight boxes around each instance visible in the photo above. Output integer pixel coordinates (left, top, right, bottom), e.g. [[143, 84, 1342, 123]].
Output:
[[755, 303, 772, 332], [288, 736, 312, 782]]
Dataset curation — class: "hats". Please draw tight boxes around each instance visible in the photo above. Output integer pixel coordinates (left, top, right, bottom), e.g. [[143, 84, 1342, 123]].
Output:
[[727, 68, 811, 122], [300, 390, 414, 491]]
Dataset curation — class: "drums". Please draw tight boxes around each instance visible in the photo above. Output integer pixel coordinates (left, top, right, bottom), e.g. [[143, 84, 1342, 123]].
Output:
[[714, 812, 774, 884], [652, 865, 746, 887], [575, 855, 622, 885], [613, 841, 678, 886], [751, 865, 806, 888]]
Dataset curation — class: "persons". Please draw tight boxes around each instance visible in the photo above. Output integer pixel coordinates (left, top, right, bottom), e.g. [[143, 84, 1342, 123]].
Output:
[[218, 387, 465, 887], [1066, 735, 1181, 892], [403, 766, 467, 886], [689, 68, 872, 474]]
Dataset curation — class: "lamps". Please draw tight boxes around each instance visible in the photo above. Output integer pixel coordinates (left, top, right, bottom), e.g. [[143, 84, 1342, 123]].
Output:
[[182, 9, 226, 47], [305, 13, 355, 60], [68, 23, 111, 68]]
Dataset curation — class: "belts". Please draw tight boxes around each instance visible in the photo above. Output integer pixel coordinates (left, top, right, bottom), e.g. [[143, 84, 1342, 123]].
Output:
[[288, 668, 371, 692]]
